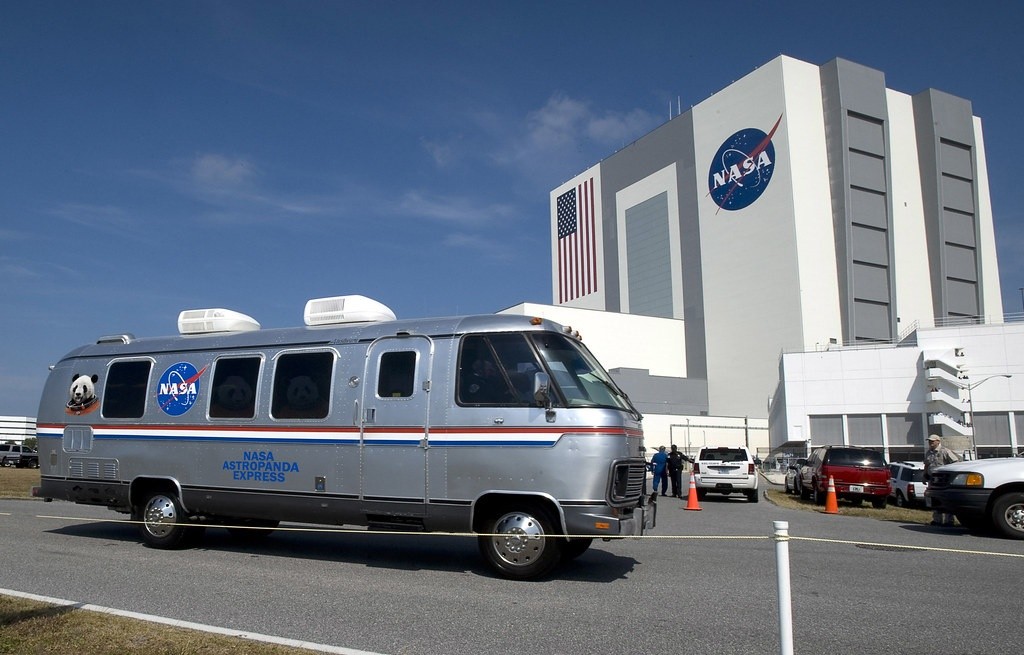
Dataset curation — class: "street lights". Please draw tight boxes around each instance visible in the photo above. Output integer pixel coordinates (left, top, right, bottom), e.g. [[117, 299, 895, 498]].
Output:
[[968, 374, 1012, 460]]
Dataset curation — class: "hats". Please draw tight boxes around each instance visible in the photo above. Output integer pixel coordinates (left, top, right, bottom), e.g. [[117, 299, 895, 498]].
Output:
[[925, 434, 940, 442]]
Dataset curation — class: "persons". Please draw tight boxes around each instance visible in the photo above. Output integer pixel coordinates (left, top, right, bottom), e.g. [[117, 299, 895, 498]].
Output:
[[650, 446, 669, 496], [666, 444, 694, 498], [921, 434, 959, 525]]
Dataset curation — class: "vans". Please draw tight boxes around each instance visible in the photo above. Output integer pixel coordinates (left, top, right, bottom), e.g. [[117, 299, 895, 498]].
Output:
[[0, 443, 33, 464]]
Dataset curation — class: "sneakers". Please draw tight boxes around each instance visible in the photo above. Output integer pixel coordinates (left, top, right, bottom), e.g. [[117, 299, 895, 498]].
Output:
[[672, 494, 676, 497], [662, 494, 667, 496], [679, 495, 682, 498]]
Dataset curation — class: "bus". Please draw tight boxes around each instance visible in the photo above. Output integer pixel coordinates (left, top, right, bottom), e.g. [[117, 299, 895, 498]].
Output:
[[32, 314, 656, 581]]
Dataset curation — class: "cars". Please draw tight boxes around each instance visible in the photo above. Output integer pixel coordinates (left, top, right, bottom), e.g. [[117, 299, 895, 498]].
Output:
[[924, 452, 1024, 540], [784, 458, 802, 495]]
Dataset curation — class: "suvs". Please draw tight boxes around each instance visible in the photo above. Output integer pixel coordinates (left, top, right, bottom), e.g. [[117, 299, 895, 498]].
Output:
[[796, 445, 892, 509], [692, 445, 761, 503], [887, 461, 928, 507]]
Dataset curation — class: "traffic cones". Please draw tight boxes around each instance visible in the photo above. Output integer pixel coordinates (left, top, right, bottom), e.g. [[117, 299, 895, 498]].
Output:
[[682, 472, 703, 511], [820, 475, 842, 514]]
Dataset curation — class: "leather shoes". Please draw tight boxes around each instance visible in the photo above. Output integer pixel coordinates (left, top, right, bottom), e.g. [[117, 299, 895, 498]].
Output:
[[943, 522, 954, 527], [931, 521, 942, 526]]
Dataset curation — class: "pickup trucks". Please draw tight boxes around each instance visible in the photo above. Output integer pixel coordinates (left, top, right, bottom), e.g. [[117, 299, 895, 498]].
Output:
[[1, 446, 38, 470]]
